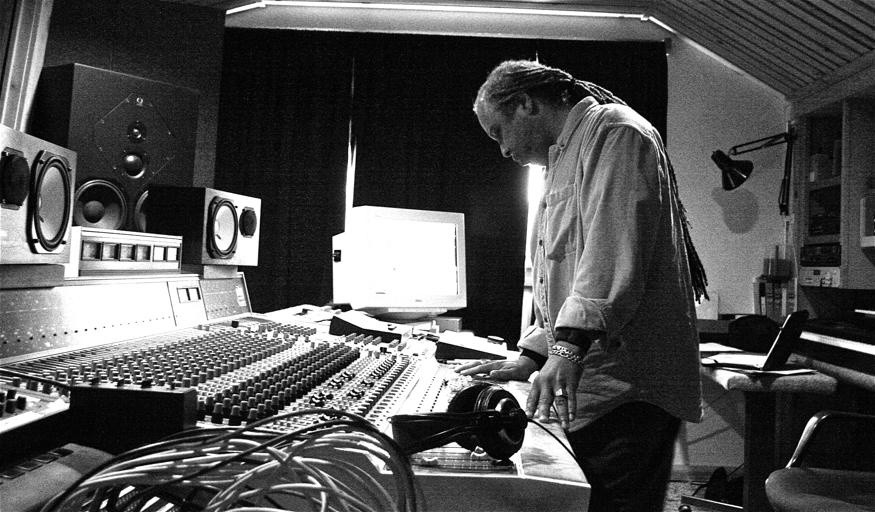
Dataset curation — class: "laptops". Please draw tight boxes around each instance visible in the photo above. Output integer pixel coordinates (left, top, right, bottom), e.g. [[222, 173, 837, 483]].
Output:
[[700, 309, 810, 372]]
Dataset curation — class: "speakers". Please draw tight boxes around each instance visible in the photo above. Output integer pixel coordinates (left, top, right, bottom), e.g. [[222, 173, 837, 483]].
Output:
[[25, 61, 200, 231], [146, 184, 262, 266], [0, 122, 78, 264]]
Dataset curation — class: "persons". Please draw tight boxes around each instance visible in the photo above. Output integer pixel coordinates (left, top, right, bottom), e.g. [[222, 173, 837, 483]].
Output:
[[445, 59, 712, 512]]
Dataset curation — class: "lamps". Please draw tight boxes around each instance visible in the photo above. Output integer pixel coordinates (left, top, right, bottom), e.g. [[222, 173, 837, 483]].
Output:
[[712, 129, 798, 320]]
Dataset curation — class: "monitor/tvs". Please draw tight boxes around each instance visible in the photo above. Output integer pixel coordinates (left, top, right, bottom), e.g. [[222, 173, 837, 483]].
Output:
[[332, 204, 467, 322]]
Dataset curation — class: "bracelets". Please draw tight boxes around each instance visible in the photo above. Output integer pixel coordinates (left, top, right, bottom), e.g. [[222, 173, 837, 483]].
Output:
[[551, 345, 585, 370]]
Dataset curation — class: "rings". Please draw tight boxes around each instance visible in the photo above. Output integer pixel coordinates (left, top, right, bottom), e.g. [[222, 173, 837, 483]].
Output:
[[555, 388, 569, 399]]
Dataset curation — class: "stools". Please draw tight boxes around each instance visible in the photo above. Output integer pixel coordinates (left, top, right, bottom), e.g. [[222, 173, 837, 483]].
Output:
[[762, 406, 873, 512]]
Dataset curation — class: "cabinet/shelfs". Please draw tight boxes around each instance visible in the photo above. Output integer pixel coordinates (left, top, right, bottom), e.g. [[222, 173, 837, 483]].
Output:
[[783, 56, 875, 292]]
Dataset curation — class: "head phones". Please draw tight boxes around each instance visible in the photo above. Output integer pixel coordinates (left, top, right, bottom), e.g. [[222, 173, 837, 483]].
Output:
[[390, 382, 527, 462]]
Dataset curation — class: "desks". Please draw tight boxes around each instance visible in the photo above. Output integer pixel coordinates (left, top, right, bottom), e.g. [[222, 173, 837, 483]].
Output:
[[687, 344, 837, 511]]
